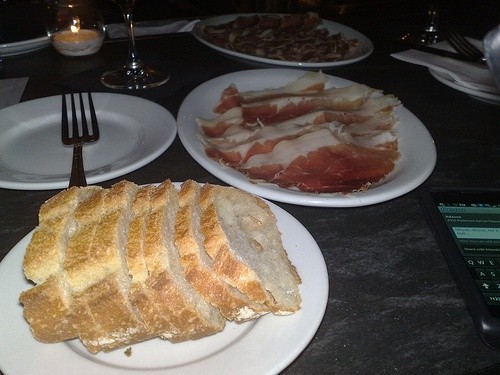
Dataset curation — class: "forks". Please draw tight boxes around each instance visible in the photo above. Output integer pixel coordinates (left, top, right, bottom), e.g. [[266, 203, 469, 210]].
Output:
[[436, 19, 488, 65], [61, 91, 100, 187]]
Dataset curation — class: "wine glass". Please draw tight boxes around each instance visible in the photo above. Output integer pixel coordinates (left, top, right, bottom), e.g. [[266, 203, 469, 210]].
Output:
[[99, 0, 171, 90]]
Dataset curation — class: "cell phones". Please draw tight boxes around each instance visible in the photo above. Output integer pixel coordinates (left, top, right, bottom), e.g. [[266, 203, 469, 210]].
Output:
[[426, 186, 500, 352]]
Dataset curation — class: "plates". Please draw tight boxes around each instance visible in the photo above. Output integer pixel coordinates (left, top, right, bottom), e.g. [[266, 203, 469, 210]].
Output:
[[192, 14, 375, 69], [0, 35, 48, 57], [0, 182, 329, 375], [0, 93, 177, 190], [177, 68, 437, 207], [427, 66, 500, 106]]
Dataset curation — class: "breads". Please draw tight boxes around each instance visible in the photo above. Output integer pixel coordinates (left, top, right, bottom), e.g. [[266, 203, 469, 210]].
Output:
[[19, 179, 303, 353]]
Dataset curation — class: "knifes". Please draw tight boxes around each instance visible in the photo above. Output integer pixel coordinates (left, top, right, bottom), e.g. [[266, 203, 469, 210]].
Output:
[[387, 35, 488, 69]]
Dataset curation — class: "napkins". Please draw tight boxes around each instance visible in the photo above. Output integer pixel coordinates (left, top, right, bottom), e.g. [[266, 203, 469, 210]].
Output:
[[105, 17, 202, 38], [391, 37, 499, 94]]
